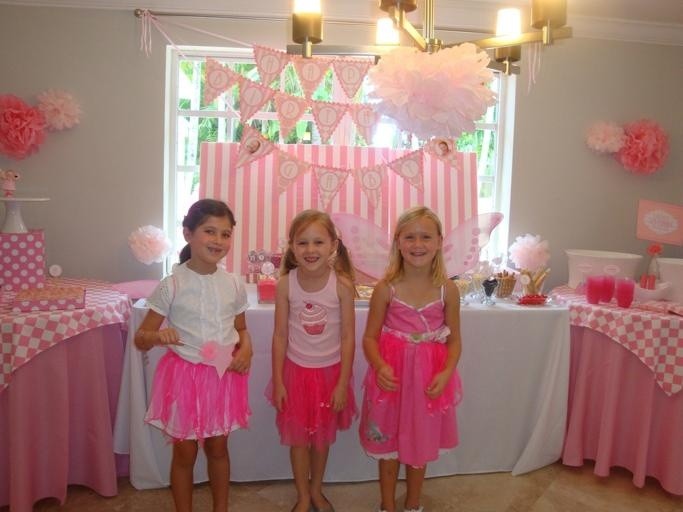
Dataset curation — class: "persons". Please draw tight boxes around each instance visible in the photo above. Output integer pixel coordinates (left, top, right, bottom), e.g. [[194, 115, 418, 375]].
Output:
[[134, 200, 252, 511], [246, 140, 260, 153], [272, 210, 355, 511], [439, 141, 450, 154], [362, 207, 461, 511]]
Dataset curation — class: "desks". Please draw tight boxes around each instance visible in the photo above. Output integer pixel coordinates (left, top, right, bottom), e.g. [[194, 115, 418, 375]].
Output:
[[113, 284, 570, 491], [0, 280, 134, 512], [548, 283, 683, 496]]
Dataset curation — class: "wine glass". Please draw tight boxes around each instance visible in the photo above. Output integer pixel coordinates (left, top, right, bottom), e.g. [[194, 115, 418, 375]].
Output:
[[454, 282, 468, 307], [470, 277, 496, 306]]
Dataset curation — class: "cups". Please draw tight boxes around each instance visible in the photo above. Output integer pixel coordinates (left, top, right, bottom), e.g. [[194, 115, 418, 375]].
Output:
[[585, 275, 603, 304], [603, 274, 616, 303], [617, 279, 634, 308]]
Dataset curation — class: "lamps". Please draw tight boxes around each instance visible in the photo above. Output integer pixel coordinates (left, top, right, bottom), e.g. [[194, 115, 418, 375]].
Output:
[[375, 17, 401, 47], [494, 9, 521, 61], [531, 1, 567, 29], [292, 0, 324, 43], [291, 1, 572, 76]]
